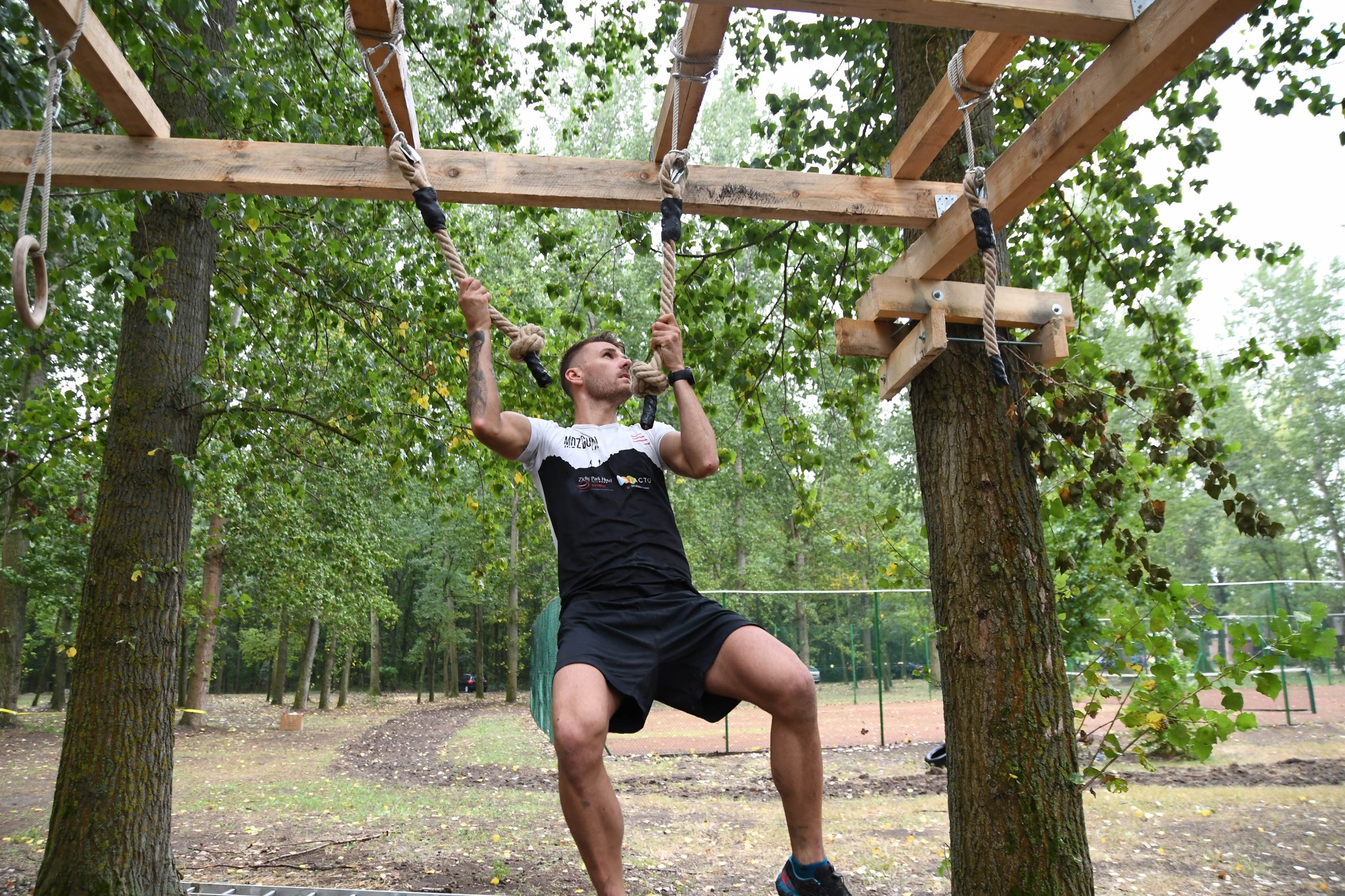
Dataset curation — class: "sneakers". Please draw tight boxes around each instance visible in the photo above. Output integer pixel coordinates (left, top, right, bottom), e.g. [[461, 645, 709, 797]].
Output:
[[776, 856, 851, 896]]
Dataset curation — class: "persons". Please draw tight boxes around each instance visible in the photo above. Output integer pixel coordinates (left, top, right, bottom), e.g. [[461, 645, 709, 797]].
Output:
[[458, 276, 854, 896]]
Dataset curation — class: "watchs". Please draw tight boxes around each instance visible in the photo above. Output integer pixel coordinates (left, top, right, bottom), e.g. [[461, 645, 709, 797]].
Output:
[[668, 366, 696, 387]]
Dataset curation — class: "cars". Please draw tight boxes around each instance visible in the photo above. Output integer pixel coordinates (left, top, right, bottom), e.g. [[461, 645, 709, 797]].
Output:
[[808, 665, 821, 684], [891, 662, 924, 680], [458, 673, 488, 693], [1100, 636, 1146, 673], [828, 660, 862, 681]]
